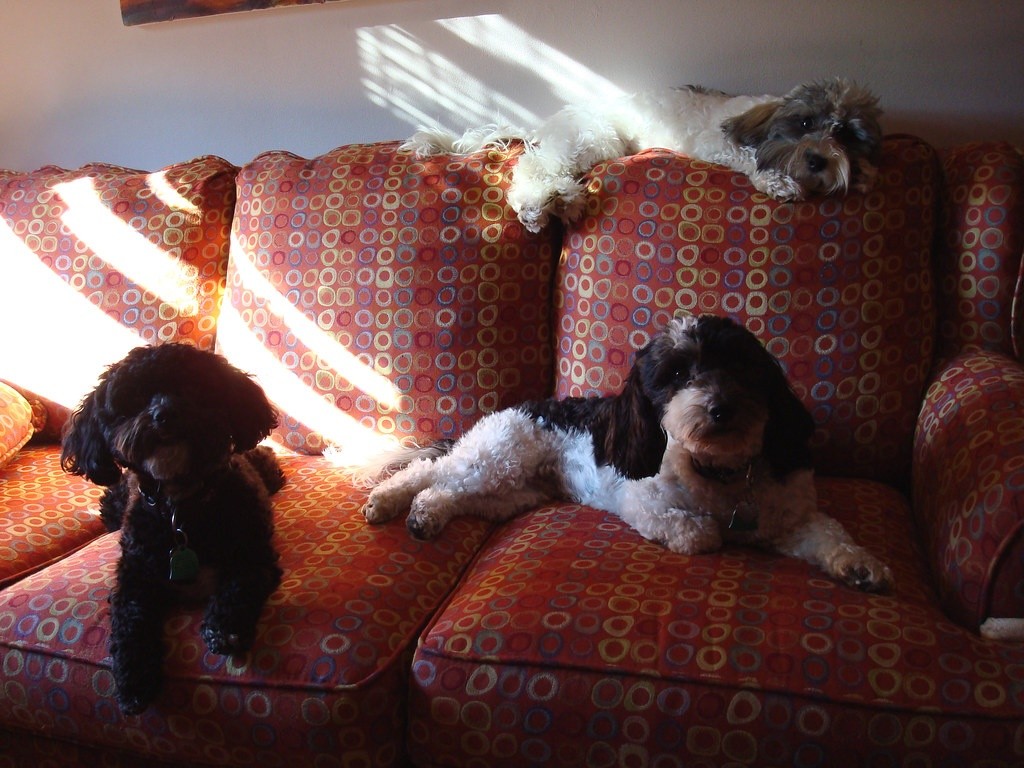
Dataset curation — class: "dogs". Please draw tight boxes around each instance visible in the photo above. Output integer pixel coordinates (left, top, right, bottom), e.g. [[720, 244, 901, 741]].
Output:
[[318, 310, 891, 599], [60, 339, 287, 717], [396, 76, 891, 235]]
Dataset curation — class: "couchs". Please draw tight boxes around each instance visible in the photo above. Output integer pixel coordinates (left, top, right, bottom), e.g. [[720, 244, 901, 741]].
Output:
[[0, 133, 1024, 768]]
